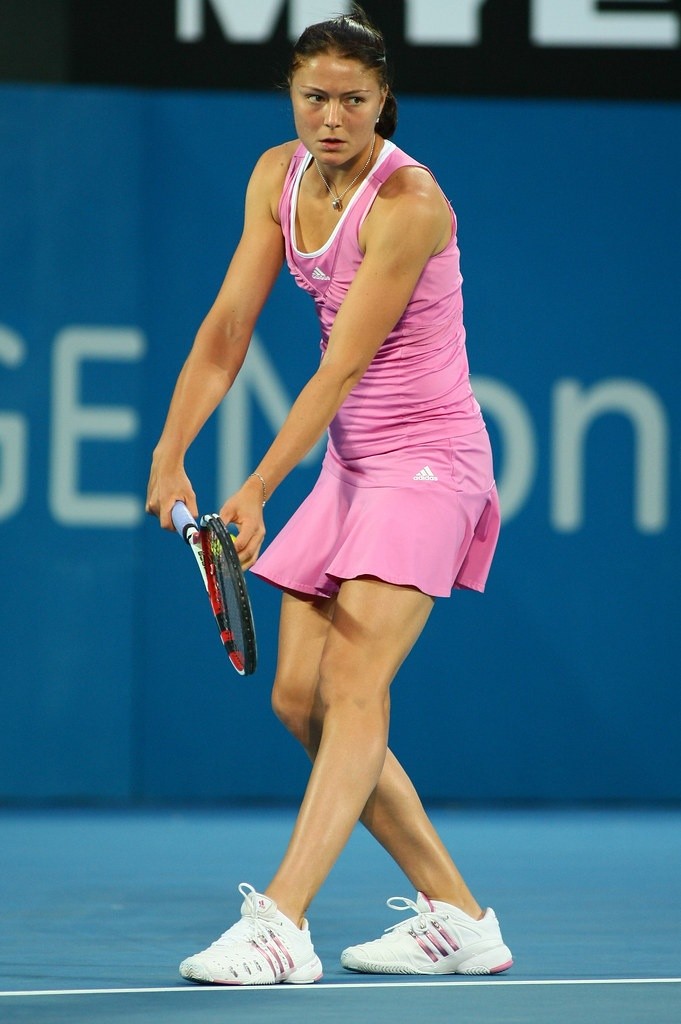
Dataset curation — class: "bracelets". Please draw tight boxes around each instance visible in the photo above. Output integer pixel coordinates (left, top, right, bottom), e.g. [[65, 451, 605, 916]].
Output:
[[250, 473, 267, 509]]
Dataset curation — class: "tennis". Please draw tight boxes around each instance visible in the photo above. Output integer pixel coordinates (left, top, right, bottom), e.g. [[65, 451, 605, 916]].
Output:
[[210, 522, 240, 560]]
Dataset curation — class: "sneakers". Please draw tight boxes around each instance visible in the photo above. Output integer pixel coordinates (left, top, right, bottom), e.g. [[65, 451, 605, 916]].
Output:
[[340, 888, 515, 976], [179, 882, 325, 985]]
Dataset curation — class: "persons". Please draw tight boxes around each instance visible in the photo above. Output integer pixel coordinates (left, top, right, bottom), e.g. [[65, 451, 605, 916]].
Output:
[[146, 5, 513, 983]]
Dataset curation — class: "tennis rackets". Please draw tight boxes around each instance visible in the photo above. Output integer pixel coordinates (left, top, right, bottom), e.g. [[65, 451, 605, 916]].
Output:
[[170, 501, 258, 676]]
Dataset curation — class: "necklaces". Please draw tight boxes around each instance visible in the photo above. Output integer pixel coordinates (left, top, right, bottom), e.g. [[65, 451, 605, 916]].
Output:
[[314, 138, 375, 210]]
